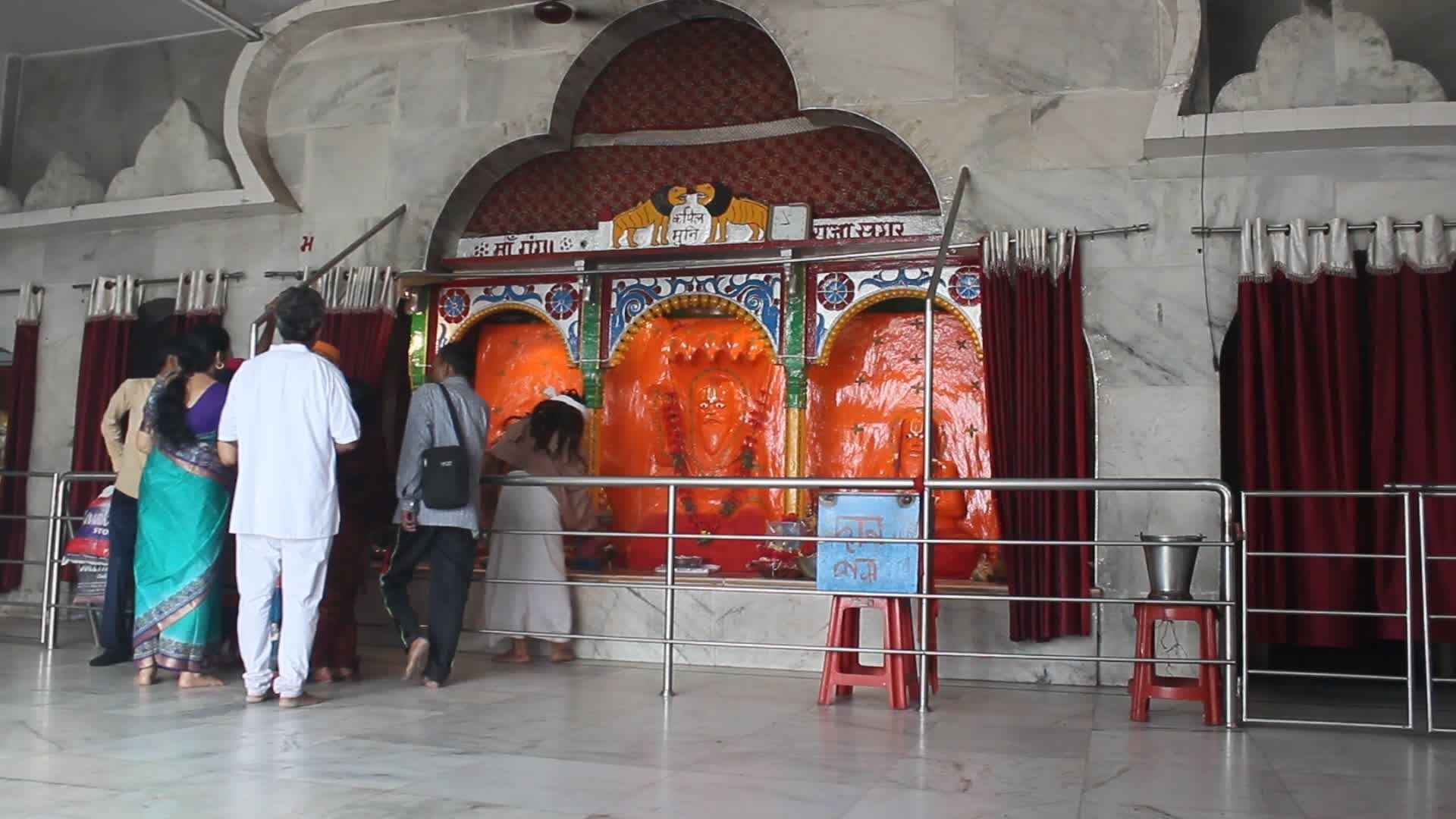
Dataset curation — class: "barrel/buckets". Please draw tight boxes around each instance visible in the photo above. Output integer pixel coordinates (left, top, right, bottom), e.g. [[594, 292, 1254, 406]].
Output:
[[1135, 531, 1207, 599]]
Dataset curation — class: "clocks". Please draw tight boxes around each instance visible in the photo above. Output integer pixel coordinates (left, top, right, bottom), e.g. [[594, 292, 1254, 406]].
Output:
[[767, 202, 811, 242]]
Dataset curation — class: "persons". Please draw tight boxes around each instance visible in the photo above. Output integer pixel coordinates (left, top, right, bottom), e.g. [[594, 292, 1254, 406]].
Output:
[[90, 322, 238, 688], [378, 343, 488, 689], [217, 285, 361, 707], [484, 388, 586, 662], [636, 369, 770, 573], [891, 413, 978, 580]]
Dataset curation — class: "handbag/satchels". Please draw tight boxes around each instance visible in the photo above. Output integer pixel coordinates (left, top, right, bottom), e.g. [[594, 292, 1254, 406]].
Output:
[[419, 445, 472, 509]]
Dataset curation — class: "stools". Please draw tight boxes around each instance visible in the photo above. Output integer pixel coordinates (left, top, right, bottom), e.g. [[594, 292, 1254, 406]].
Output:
[[1127, 601, 1227, 725], [818, 595, 939, 709]]
